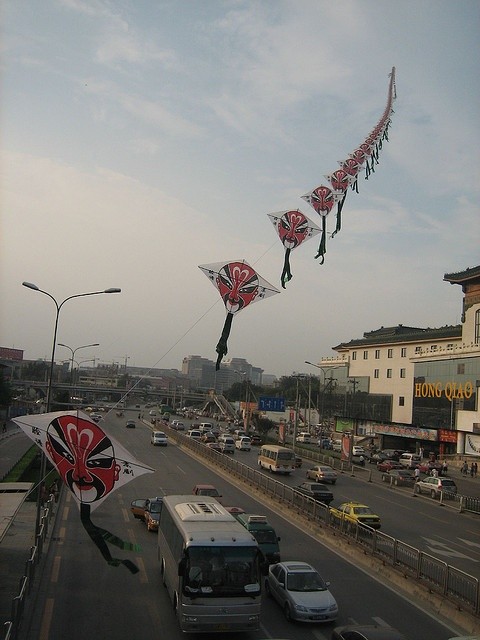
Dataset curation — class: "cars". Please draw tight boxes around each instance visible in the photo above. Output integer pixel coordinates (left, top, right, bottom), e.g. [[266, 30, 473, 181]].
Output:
[[201, 432, 216, 442], [171, 420, 179, 428], [292, 482, 334, 505], [149, 410, 155, 415], [205, 442, 220, 452], [126, 420, 136, 429], [306, 465, 338, 485], [352, 445, 365, 457], [377, 459, 403, 471], [333, 624, 407, 640], [192, 485, 222, 504], [367, 452, 389, 464], [176, 422, 184, 430], [90, 417, 100, 421], [136, 404, 140, 408], [329, 502, 381, 533], [251, 434, 261, 445], [295, 454, 302, 468], [129, 497, 163, 531], [265, 561, 338, 623], [332, 440, 341, 450], [218, 434, 230, 441], [382, 470, 411, 486], [223, 506, 245, 518]]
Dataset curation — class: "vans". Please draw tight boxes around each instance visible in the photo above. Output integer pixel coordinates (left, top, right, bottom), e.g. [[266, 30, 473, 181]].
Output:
[[237, 513, 281, 575], [398, 453, 421, 470], [199, 423, 212, 432], [235, 436, 252, 451], [296, 432, 311, 444], [151, 430, 168, 446], [219, 437, 235, 454], [233, 429, 245, 440]]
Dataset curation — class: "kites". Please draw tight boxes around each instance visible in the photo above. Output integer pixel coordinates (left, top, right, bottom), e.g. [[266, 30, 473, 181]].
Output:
[[197, 258, 281, 372], [300, 184, 345, 264], [8, 410, 157, 575], [266, 208, 324, 290], [337, 156, 365, 191], [346, 66, 399, 179], [323, 168, 357, 237]]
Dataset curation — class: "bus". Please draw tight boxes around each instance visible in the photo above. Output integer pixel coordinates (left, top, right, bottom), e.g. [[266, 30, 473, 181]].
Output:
[[158, 494, 269, 633], [257, 445, 296, 475], [160, 404, 172, 414]]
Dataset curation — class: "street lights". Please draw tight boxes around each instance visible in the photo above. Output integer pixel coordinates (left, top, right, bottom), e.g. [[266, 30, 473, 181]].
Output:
[[22, 282, 121, 546], [58, 344, 99, 410], [305, 361, 339, 453], [234, 370, 247, 414], [70, 359, 94, 386]]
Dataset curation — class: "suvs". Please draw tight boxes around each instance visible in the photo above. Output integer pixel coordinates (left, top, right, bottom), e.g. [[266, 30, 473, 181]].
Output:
[[190, 430, 203, 441], [89, 412, 102, 417], [413, 477, 457, 499]]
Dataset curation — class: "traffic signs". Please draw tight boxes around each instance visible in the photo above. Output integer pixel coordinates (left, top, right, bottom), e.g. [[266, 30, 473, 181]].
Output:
[[258, 397, 285, 412]]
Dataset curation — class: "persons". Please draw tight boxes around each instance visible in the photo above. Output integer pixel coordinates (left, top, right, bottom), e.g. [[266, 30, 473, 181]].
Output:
[[411, 459, 480, 480]]
